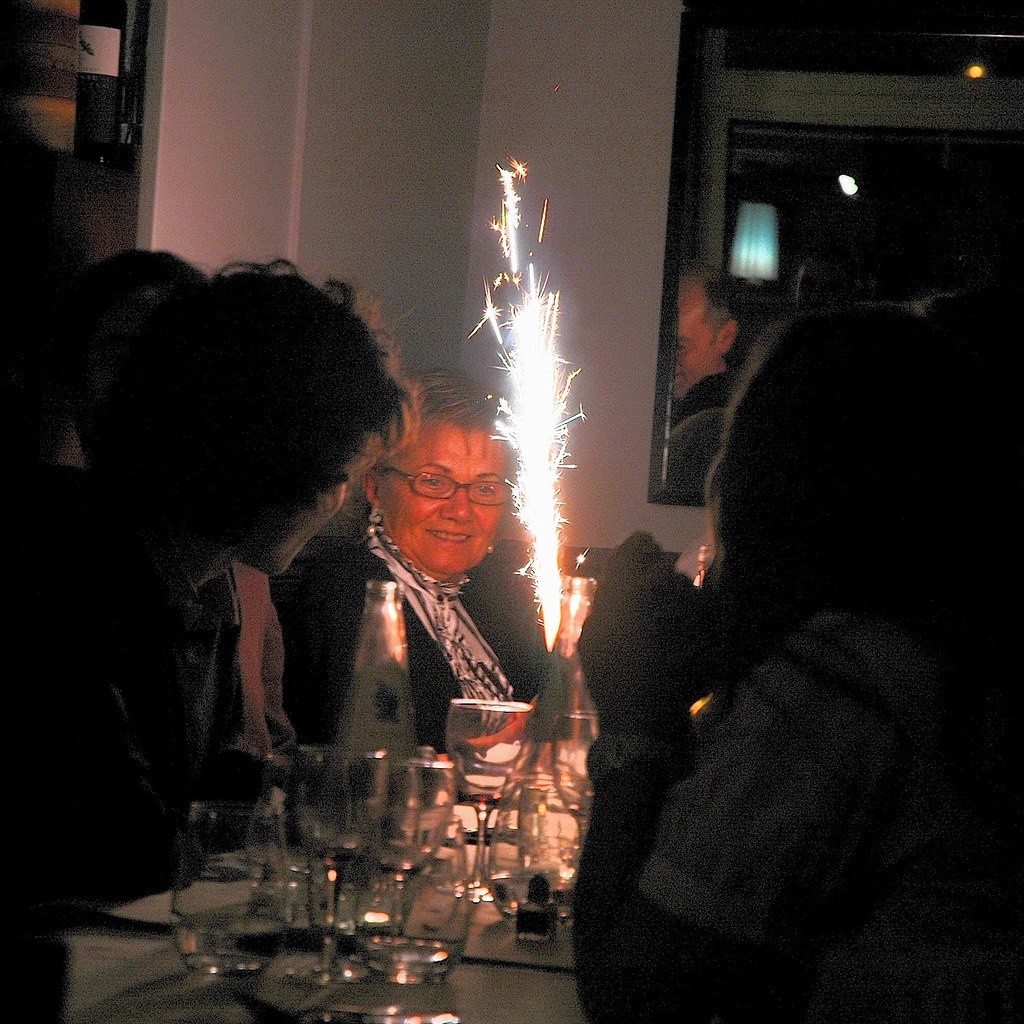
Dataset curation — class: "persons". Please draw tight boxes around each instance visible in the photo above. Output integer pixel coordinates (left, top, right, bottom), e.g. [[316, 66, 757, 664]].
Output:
[[576, 305, 1024, 1024], [0, 250, 418, 1023], [265, 370, 568, 844], [663, 253, 746, 501]]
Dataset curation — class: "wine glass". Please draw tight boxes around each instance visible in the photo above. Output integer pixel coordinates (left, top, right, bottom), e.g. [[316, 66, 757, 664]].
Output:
[[444, 699, 534, 903], [553, 711, 615, 860], [252, 746, 456, 1012]]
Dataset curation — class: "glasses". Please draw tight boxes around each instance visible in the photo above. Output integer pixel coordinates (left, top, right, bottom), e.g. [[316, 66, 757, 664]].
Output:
[[392, 469, 512, 505]]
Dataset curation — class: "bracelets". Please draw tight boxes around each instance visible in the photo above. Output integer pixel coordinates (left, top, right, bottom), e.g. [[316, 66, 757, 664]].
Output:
[[587, 715, 692, 782]]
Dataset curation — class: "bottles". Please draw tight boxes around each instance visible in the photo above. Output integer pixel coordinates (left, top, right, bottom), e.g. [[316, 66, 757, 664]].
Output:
[[306, 579, 414, 935], [689, 546, 715, 589], [512, 876, 559, 946], [487, 575, 603, 922]]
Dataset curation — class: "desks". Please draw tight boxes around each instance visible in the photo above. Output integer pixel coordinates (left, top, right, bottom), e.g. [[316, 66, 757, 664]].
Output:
[[58, 861, 593, 1024]]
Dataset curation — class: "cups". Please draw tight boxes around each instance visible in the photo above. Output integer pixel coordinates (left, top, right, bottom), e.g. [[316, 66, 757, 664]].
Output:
[[355, 820, 468, 984], [173, 800, 288, 978], [521, 781, 576, 875]]
